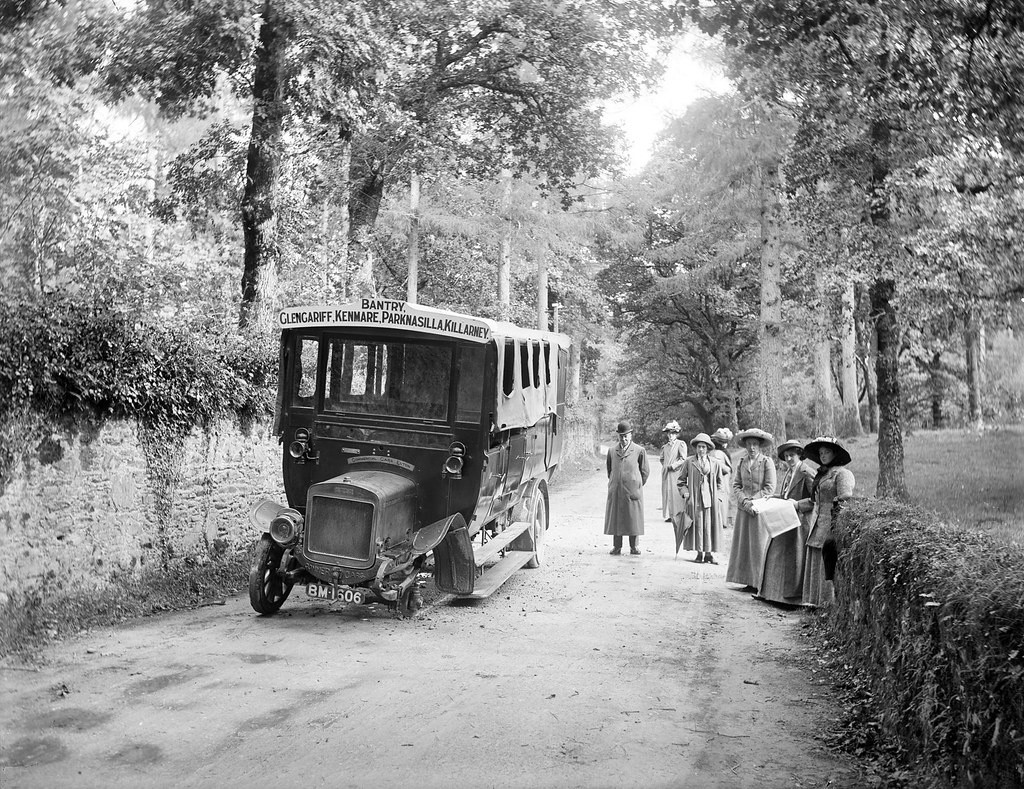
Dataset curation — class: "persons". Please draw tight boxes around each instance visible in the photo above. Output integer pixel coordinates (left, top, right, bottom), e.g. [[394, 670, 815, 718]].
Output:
[[803, 435, 855, 607], [606, 421, 650, 555], [660, 420, 687, 521], [726, 428, 777, 592], [751, 439, 820, 606], [676, 433, 725, 565], [710, 428, 734, 528]]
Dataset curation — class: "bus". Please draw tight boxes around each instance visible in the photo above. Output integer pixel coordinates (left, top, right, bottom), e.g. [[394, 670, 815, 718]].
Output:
[[248, 298, 571, 615]]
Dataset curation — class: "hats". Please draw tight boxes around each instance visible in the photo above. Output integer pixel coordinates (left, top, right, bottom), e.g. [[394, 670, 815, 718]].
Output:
[[662, 420, 682, 433], [690, 432, 715, 452], [777, 440, 806, 461], [734, 427, 774, 448], [803, 435, 852, 467], [616, 421, 633, 435], [710, 427, 733, 443]]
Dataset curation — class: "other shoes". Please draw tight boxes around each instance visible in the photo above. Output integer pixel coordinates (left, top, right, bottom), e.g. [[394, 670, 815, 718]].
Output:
[[751, 593, 760, 599], [630, 548, 641, 554], [610, 548, 621, 555], [695, 555, 718, 565]]
